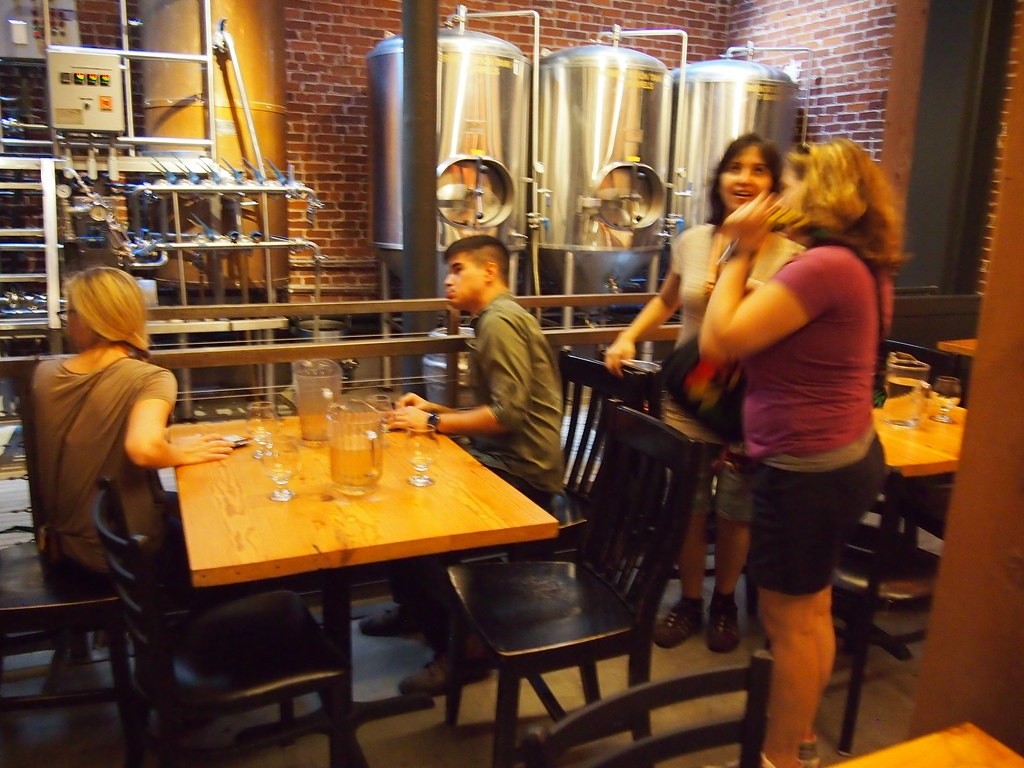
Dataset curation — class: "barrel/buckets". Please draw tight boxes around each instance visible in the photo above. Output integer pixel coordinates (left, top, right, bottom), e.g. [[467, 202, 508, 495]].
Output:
[[422, 325, 478, 410], [296, 319, 348, 342]]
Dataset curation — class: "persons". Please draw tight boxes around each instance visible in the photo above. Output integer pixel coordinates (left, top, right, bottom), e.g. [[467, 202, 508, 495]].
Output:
[[360, 235, 564, 693], [698, 140, 893, 768], [603, 133, 785, 649], [34, 269, 234, 697]]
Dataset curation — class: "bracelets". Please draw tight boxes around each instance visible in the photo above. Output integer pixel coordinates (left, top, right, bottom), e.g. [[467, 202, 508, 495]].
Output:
[[727, 238, 759, 262]]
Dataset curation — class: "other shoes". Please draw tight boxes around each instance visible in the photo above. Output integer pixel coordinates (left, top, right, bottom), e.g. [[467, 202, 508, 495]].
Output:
[[398, 647, 491, 697], [797, 735, 820, 768], [358, 603, 426, 639]]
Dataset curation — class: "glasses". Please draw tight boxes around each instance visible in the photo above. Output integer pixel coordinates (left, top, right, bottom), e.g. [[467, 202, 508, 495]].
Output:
[[57, 306, 77, 335]]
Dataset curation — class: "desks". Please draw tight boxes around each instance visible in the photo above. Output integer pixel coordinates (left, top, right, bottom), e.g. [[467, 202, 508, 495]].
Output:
[[170, 409, 561, 765], [819, 719, 1023, 767], [865, 387, 968, 577], [935, 337, 977, 357]]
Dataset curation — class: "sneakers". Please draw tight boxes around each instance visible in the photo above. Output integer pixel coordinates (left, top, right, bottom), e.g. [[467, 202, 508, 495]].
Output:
[[653, 603, 705, 649], [706, 594, 741, 654]]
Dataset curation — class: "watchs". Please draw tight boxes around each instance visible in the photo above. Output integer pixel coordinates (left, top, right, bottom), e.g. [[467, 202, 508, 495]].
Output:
[[428, 411, 440, 433]]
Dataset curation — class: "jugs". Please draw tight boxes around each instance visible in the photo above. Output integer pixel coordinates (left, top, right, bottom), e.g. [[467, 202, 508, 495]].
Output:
[[291, 358, 343, 448], [881, 352, 933, 429]]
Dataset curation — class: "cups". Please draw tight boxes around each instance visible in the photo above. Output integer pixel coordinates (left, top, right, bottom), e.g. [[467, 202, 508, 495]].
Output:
[[324, 400, 384, 496]]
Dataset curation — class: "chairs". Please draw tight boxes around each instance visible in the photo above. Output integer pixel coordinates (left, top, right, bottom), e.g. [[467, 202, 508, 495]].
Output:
[[92, 472, 369, 768], [448, 343, 651, 564], [832, 469, 939, 758], [441, 395, 702, 768], [0, 540, 144, 768], [544, 647, 774, 768]]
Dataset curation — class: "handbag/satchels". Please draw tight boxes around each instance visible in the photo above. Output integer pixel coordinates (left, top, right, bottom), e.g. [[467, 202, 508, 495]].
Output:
[[658, 334, 747, 451]]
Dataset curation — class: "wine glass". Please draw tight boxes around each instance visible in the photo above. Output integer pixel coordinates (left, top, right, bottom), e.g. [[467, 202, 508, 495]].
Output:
[[246, 401, 279, 461], [932, 376, 962, 425], [404, 424, 441, 488], [260, 435, 303, 502], [365, 394, 394, 449]]
